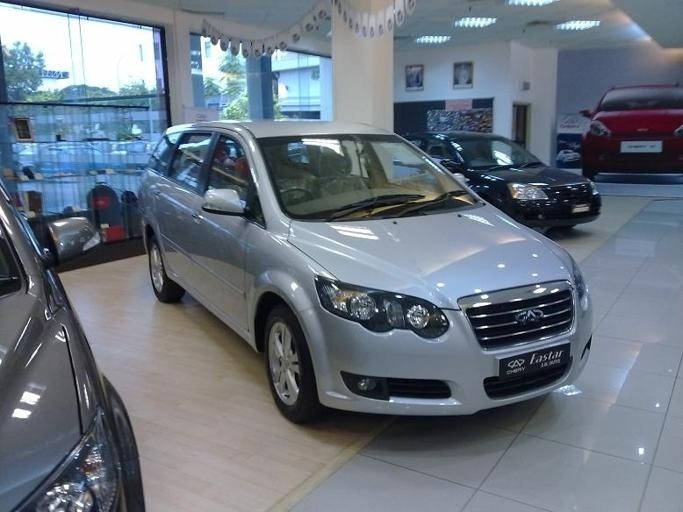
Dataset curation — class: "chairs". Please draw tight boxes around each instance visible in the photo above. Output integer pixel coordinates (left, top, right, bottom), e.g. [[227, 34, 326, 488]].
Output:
[[304, 142, 369, 200]]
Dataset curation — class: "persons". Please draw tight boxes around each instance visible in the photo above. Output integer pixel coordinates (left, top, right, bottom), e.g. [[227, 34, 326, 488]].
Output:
[[85, 172, 120, 227]]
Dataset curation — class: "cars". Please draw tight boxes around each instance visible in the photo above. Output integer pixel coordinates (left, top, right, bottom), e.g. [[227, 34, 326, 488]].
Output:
[[579, 83, 682, 180], [0, 180, 146, 511], [138, 117, 593, 423], [402, 131, 602, 237], [12, 140, 159, 177]]
